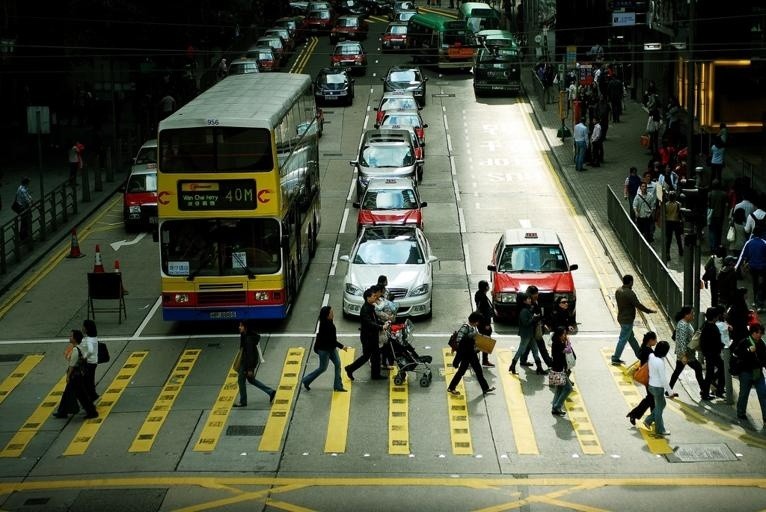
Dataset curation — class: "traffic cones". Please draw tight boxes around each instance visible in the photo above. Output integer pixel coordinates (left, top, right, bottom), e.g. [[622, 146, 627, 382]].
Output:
[[94, 244, 106, 272], [112, 260, 129, 296], [66, 224, 86, 257]]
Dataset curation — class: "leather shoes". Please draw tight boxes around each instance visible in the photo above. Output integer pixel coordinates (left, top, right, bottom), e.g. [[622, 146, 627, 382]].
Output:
[[371, 375, 388, 379], [345, 366, 354, 380]]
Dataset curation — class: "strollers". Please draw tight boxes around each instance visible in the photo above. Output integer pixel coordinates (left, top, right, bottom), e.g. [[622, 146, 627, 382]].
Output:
[[383, 318, 433, 387]]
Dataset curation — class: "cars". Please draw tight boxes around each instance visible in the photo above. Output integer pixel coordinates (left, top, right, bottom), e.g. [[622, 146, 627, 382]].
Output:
[[131, 138, 158, 165], [220, 0, 372, 107], [487, 228, 578, 323], [338, 225, 437, 323], [373, 89, 423, 128], [374, 109, 428, 147], [381, 63, 429, 105], [124, 163, 158, 233], [372, 1, 418, 53], [376, 124, 426, 180], [354, 178, 428, 234]]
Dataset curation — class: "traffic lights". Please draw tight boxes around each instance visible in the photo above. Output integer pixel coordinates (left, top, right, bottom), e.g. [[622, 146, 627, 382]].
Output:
[[680, 188, 700, 224]]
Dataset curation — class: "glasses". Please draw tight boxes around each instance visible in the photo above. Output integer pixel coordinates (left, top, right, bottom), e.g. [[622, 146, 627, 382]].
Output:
[[560, 301, 568, 304]]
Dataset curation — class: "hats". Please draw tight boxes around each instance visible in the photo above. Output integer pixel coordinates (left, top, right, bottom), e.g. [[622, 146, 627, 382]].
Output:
[[724, 256, 738, 265]]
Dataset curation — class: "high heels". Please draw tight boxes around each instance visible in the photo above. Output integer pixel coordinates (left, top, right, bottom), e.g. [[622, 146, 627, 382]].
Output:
[[626, 412, 635, 425]]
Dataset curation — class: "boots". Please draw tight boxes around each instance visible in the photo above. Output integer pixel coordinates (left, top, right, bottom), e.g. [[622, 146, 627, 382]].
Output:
[[509, 359, 518, 374], [535, 360, 547, 374]]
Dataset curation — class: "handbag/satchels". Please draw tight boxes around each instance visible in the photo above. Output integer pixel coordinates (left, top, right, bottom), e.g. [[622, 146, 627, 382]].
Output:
[[565, 351, 575, 369], [475, 330, 496, 354], [98, 343, 109, 363], [67, 359, 87, 392], [448, 330, 458, 352], [727, 226, 735, 242], [548, 369, 566, 386], [687, 329, 702, 350], [623, 361, 649, 387]]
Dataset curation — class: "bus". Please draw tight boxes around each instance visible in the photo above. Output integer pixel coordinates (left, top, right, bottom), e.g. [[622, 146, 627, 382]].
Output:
[[472, 29, 523, 95], [457, 2, 501, 30], [406, 12, 475, 73]]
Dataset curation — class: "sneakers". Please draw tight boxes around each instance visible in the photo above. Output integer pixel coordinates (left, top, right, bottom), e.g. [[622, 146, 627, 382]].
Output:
[[665, 391, 678, 397], [448, 387, 460, 394], [612, 358, 623, 364], [53, 394, 99, 419], [643, 420, 671, 435], [552, 404, 565, 414], [482, 361, 495, 367], [483, 387, 496, 394]]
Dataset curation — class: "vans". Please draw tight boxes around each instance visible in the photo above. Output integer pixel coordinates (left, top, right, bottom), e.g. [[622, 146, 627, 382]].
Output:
[[349, 129, 421, 195]]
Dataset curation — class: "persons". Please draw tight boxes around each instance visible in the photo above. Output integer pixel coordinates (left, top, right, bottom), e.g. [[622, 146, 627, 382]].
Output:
[[159, 94, 177, 116], [16, 176, 37, 244], [475, 280, 494, 367], [551, 327, 573, 416], [447, 313, 496, 393], [345, 275, 396, 379], [509, 286, 575, 388], [518, 42, 625, 172], [68, 140, 83, 186], [626, 331, 674, 435], [611, 275, 657, 364], [624, 80, 766, 340], [665, 306, 766, 426], [233, 317, 276, 407], [66, 319, 99, 412], [55, 329, 98, 419], [301, 305, 348, 392]]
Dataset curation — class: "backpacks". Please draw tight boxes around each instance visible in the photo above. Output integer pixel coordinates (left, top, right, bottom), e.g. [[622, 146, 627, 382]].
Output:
[[750, 213, 766, 237]]
